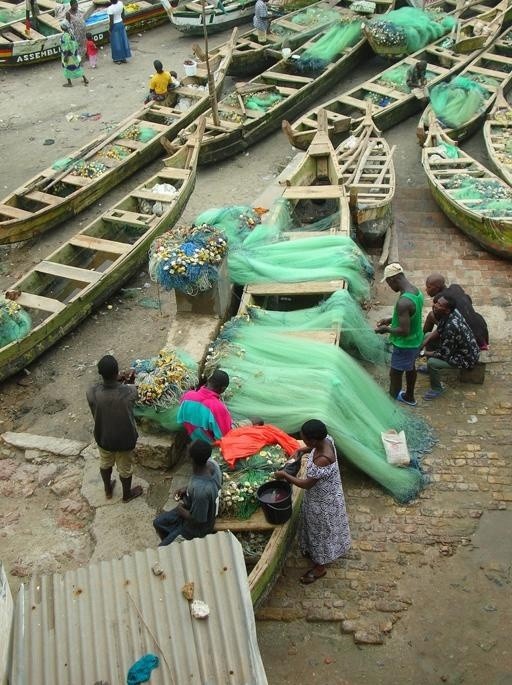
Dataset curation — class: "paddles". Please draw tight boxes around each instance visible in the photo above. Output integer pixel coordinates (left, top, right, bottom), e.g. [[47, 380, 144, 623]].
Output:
[[43, 119, 138, 191], [369, 145, 397, 192]]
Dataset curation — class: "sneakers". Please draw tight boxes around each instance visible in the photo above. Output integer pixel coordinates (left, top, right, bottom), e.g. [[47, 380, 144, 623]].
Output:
[[424, 388, 442, 400], [417, 365, 428, 373]]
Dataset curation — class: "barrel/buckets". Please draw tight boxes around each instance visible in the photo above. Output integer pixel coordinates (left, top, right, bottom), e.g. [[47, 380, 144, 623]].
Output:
[[257, 480, 292, 524], [183, 61, 197, 76], [283, 49, 291, 58]]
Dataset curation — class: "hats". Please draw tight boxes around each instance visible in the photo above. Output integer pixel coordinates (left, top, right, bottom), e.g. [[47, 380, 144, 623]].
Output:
[[154, 60, 162, 70], [379, 263, 403, 283]]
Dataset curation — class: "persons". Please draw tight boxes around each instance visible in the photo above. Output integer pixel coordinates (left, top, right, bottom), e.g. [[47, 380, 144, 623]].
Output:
[[152, 440, 222, 548], [274, 420, 352, 584], [145, 60, 173, 104], [86, 355, 143, 504], [253, 0, 273, 45], [59, 0, 132, 87], [418, 275, 489, 399], [374, 263, 424, 407], [176, 370, 265, 446], [405, 61, 427, 88]]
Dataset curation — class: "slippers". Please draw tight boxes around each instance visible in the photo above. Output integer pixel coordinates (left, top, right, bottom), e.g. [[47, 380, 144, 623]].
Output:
[[397, 391, 418, 405], [301, 570, 326, 584]]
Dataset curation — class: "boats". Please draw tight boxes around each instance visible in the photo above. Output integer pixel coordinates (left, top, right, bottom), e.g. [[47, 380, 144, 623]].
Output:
[[2, 120, 204, 384], [163, 107, 354, 610]]
